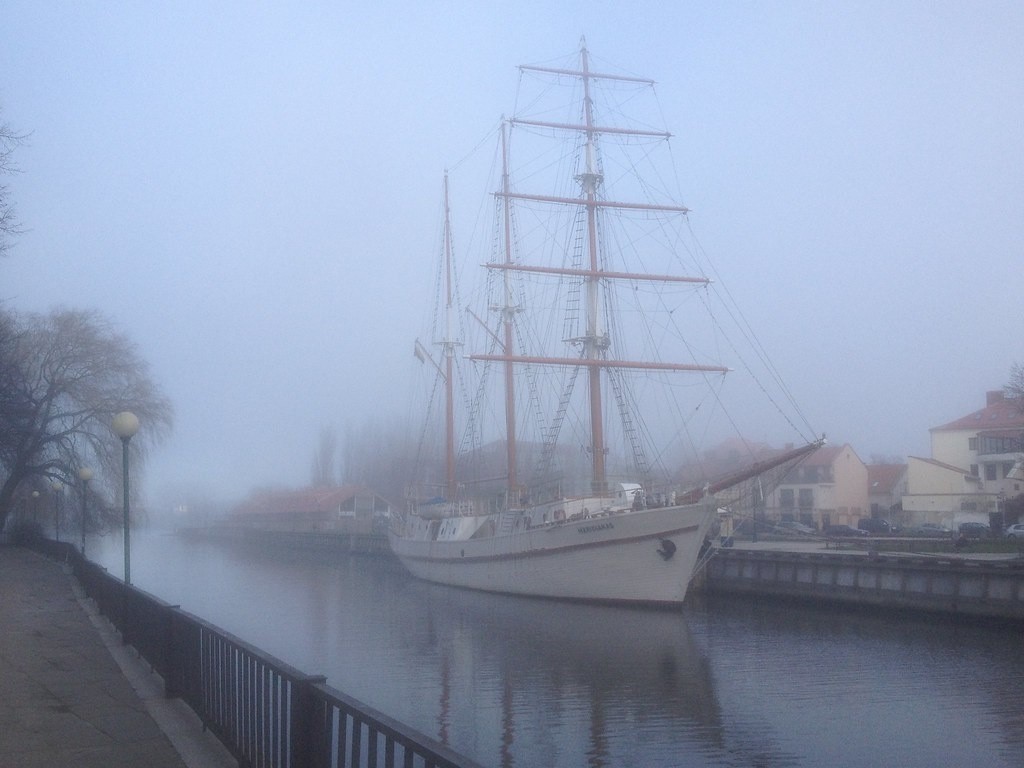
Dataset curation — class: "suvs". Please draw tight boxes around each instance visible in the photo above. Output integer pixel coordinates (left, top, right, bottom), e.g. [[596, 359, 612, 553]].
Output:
[[778, 521, 817, 538], [857, 518, 899, 536]]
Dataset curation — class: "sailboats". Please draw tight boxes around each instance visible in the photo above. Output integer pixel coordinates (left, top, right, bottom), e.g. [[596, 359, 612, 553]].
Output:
[[382, 26, 827, 613]]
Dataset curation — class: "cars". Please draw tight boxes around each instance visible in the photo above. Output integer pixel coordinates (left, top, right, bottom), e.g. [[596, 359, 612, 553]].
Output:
[[914, 520, 953, 539], [828, 523, 871, 539], [1004, 524, 1024, 542], [959, 522, 991, 538]]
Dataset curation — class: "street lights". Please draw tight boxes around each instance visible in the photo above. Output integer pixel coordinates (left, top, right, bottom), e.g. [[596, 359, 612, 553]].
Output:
[[78, 467, 95, 555], [52, 482, 65, 541], [111, 411, 141, 582]]
